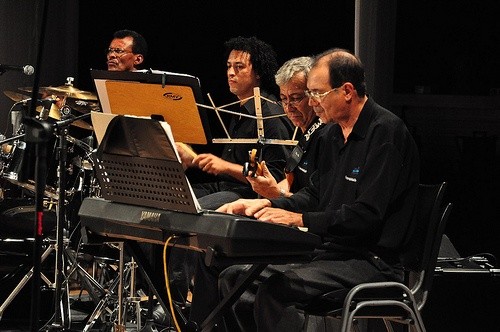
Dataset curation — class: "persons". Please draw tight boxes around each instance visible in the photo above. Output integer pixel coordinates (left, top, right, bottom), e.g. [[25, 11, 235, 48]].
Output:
[[215, 48, 412, 332], [104, 31, 333, 332]]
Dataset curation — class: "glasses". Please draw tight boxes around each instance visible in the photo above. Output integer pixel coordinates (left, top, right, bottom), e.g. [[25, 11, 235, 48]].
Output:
[[304, 82, 356, 103], [106, 47, 134, 56], [278, 95, 305, 108]]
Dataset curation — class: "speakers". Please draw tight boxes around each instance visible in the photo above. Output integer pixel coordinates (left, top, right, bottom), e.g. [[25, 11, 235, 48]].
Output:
[[420, 255, 500, 332]]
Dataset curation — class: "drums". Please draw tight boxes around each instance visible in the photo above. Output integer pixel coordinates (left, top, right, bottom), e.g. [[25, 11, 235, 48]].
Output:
[[0, 205, 57, 332], [4, 118, 92, 204], [68, 161, 120, 266]]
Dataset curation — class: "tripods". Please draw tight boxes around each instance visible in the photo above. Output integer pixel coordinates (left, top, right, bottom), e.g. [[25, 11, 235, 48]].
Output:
[[0, 178, 180, 332]]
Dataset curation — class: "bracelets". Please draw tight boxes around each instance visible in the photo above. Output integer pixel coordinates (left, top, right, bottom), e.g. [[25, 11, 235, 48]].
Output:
[[280, 189, 285, 198]]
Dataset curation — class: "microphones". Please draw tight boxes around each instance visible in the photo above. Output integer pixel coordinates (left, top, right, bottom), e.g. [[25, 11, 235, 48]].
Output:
[[0, 64, 34, 75]]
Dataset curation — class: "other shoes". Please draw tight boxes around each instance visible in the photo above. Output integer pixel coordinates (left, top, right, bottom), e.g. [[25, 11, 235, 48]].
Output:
[[178, 320, 201, 332], [151, 304, 167, 325], [71, 293, 114, 316]]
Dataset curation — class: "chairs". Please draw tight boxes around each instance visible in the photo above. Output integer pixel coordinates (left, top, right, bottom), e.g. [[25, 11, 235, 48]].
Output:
[[303, 175, 458, 332]]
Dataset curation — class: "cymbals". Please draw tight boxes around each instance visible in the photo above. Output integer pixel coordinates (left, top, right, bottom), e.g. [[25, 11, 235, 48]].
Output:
[[4, 90, 94, 130], [17, 86, 98, 101]]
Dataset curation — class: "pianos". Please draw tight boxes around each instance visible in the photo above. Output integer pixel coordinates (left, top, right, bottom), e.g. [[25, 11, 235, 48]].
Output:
[[78, 196, 322, 332]]
